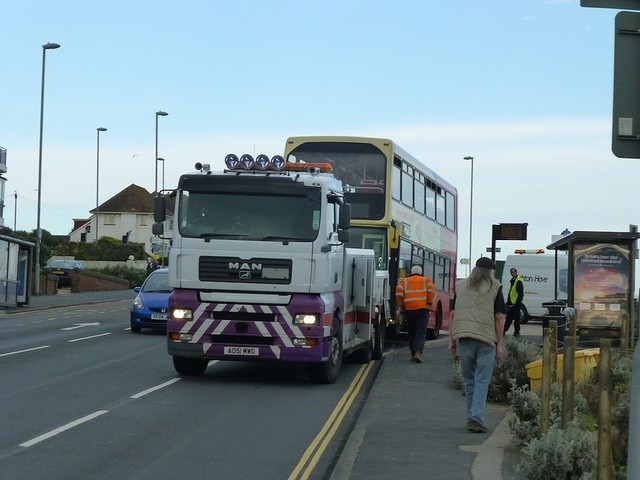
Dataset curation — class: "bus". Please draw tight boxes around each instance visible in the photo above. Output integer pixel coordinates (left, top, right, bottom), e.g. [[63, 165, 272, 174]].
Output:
[[284, 136, 458, 340]]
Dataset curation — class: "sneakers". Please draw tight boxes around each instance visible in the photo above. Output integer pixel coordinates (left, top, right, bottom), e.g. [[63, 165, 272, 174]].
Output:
[[465, 418, 488, 433], [513, 331, 520, 336], [410, 352, 423, 363]]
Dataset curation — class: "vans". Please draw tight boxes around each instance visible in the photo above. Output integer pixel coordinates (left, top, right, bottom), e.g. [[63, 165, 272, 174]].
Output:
[[502, 248, 568, 324]]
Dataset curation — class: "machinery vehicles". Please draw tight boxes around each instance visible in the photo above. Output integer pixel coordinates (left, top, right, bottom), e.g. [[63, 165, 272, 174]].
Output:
[[152, 154, 391, 384]]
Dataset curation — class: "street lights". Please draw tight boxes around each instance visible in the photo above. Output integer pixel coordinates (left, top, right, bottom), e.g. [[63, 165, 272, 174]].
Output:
[[155, 111, 169, 192], [157, 158, 165, 194], [96, 127, 108, 242], [34, 43, 60, 296], [463, 156, 474, 276]]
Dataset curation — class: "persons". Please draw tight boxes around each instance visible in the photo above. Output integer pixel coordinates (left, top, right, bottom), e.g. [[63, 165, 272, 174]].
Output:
[[503, 268, 525, 338], [395, 266, 435, 362], [447, 257, 504, 433]]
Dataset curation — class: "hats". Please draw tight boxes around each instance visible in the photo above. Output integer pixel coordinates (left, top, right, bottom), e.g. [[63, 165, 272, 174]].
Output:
[[476, 257, 497, 269]]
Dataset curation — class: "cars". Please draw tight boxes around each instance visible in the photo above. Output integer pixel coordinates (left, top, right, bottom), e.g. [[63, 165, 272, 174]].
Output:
[[130, 269, 173, 333], [46, 260, 88, 288]]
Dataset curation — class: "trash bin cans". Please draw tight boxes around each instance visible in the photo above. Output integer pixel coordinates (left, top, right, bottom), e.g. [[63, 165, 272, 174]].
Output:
[[524, 348, 600, 393], [542, 300, 566, 348]]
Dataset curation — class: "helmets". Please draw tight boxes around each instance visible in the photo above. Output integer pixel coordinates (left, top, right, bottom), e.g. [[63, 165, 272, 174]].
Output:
[[411, 265, 422, 275]]
